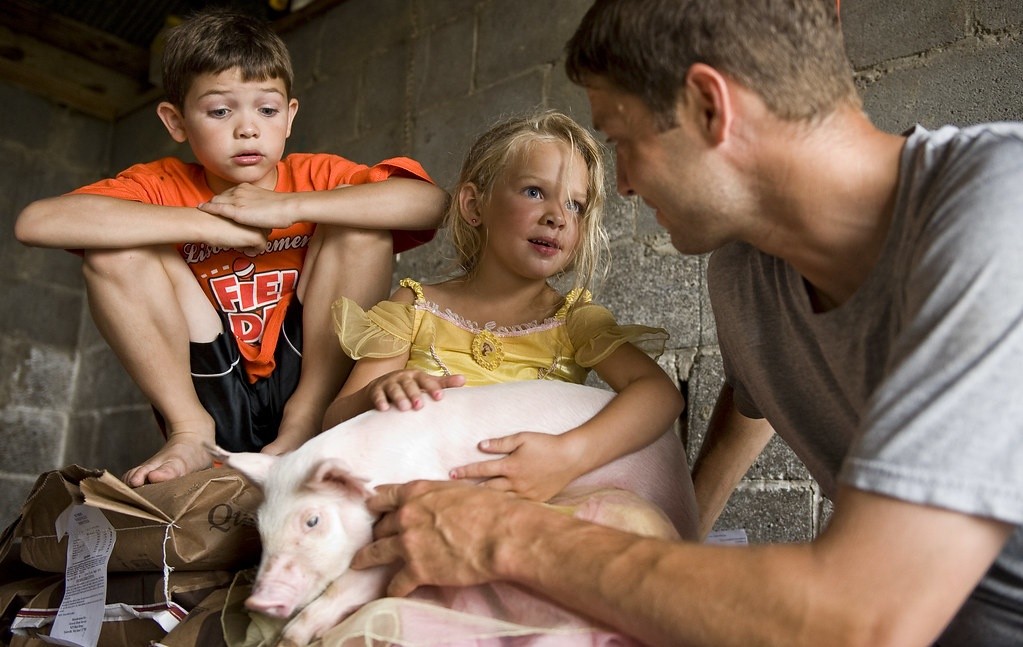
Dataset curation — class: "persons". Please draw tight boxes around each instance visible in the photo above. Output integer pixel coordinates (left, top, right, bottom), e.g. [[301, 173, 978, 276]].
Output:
[[347, 1, 1023, 645], [12, 14, 459, 504], [320, 109, 692, 521]]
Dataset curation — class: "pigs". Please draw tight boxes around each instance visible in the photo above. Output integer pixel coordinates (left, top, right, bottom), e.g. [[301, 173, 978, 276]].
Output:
[[201, 379, 702, 647]]
